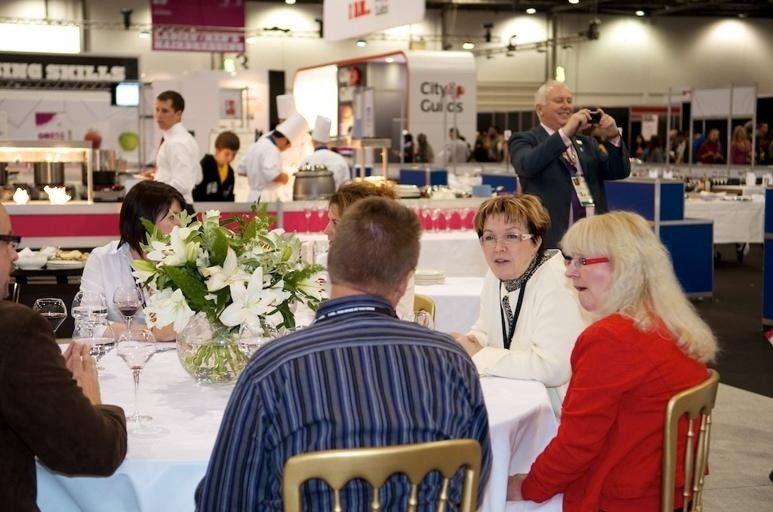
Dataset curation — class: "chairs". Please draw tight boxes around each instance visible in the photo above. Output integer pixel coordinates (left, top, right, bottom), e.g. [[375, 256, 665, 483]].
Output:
[[661, 368, 722, 512], [281, 438, 482, 512]]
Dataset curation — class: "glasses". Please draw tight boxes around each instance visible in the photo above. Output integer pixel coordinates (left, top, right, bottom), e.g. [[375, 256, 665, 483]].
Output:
[[0, 234, 21, 249], [479, 233, 534, 246], [563, 257, 609, 271]]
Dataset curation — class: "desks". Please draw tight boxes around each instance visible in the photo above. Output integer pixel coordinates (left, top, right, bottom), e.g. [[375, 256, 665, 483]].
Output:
[[414, 275, 491, 335], [22, 337, 566, 512], [627, 158, 773, 265]]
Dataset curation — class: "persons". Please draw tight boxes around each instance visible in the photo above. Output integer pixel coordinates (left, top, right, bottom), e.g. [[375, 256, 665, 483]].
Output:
[[295, 177, 415, 328], [507, 210, 723, 512], [238, 131, 290, 202], [192, 133, 240, 202], [298, 138, 350, 193], [508, 80, 631, 259], [154, 89, 203, 227], [401, 119, 773, 164], [449, 194, 591, 417], [276, 119, 315, 166], [0, 204, 127, 511], [74, 179, 187, 343], [194, 196, 493, 511]]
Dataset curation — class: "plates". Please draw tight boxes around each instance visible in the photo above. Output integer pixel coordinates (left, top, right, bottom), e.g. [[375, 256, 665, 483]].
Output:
[[47, 261, 82, 269], [414, 271, 445, 285]]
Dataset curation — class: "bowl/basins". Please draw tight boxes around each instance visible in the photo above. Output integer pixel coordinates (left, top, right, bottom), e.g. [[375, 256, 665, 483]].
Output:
[[15, 252, 47, 269], [472, 185, 492, 197], [446, 165, 483, 198]]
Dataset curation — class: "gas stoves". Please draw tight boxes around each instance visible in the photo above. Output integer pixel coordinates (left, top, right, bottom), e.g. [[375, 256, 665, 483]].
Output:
[[9, 183, 76, 200]]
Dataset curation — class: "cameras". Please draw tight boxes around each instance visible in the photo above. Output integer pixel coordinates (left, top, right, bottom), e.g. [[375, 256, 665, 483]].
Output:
[[587, 112, 601, 123]]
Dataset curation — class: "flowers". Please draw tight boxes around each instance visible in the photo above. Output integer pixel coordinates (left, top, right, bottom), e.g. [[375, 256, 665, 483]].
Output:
[[133, 191, 328, 382]]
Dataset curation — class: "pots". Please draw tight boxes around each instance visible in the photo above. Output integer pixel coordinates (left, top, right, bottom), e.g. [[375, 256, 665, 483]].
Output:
[[33, 162, 64, 186], [81, 170, 137, 187], [0, 170, 19, 185]]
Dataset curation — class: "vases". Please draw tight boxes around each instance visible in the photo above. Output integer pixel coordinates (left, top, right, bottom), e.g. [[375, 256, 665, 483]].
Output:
[[175, 314, 279, 387]]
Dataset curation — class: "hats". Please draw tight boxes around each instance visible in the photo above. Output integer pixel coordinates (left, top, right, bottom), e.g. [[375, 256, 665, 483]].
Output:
[[277, 94, 295, 119], [311, 116, 330, 144], [275, 114, 308, 149]]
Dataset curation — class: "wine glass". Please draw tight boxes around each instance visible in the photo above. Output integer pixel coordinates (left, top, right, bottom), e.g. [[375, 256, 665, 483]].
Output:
[[239, 320, 277, 359], [402, 311, 434, 329], [302, 206, 471, 235], [34, 287, 157, 423]]
